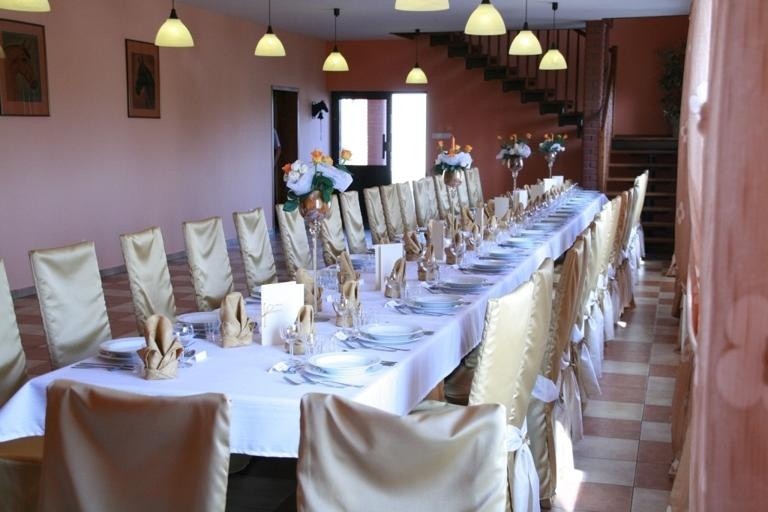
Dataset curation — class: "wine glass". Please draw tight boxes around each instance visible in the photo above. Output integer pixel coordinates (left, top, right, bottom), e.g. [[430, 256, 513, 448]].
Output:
[[173, 311, 221, 368], [312, 181, 579, 345], [280, 322, 315, 370]]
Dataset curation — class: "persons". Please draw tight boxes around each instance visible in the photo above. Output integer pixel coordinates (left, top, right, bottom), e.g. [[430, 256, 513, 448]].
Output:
[[274, 127, 283, 166]]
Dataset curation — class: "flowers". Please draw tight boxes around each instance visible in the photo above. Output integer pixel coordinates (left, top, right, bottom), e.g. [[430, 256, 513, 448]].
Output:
[[281, 150, 353, 213], [494, 132, 534, 161], [538, 132, 568, 155], [431, 135, 473, 174]]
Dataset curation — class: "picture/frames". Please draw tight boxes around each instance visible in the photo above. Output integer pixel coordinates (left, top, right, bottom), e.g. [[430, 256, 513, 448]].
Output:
[[0, 17, 51, 117], [125, 38, 161, 118]]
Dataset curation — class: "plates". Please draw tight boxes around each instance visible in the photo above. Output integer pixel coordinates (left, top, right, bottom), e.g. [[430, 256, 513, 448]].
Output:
[[97, 352, 137, 361], [303, 366, 384, 378], [100, 336, 145, 354], [310, 348, 381, 373]]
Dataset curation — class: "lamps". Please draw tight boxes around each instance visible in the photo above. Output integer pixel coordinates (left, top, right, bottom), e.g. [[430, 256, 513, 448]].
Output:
[[394, 0, 450, 13], [154, 0, 194, 48], [254, 0, 286, 57], [405, 29, 428, 85], [322, 8, 350, 72], [508, 0, 543, 56], [463, 0, 507, 36], [538, 2, 569, 71], [0, 0, 50, 12]]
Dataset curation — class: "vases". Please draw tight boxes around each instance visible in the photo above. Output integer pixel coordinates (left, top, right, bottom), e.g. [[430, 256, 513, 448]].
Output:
[[506, 155, 524, 190], [544, 151, 558, 178]]
